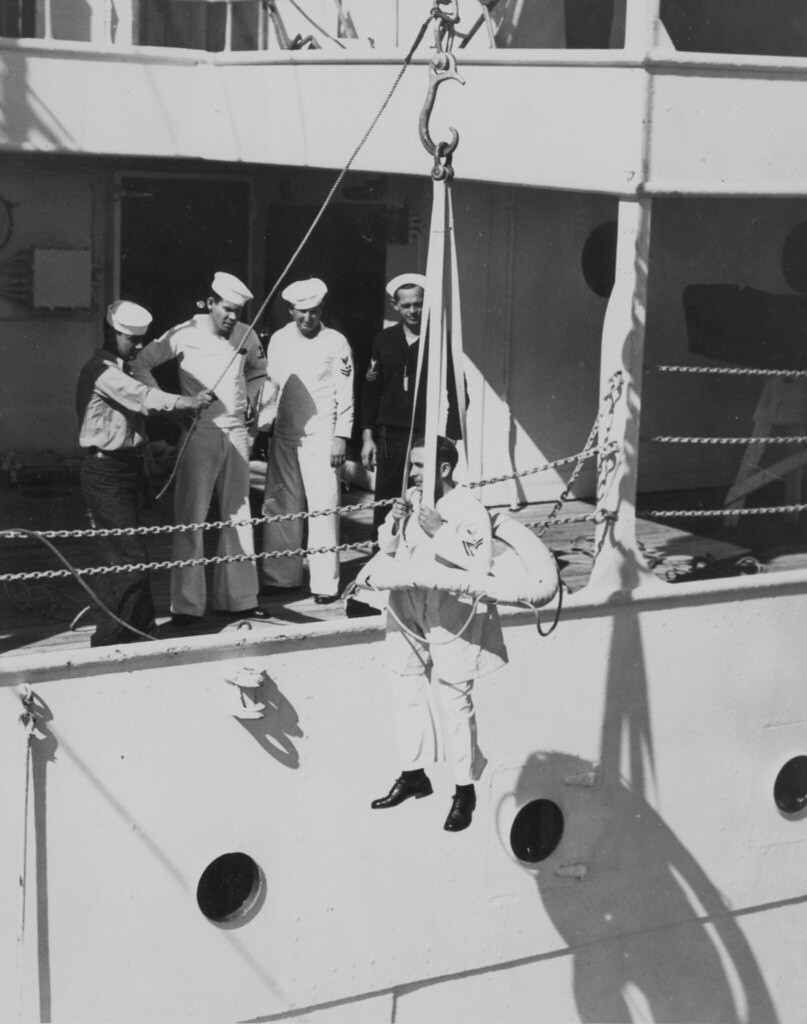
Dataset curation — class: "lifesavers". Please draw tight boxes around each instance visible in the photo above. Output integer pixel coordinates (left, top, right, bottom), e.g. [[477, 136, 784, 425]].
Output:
[[355, 509, 560, 606]]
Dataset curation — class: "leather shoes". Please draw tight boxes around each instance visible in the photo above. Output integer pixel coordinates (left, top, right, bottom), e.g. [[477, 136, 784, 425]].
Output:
[[172, 612, 193, 626], [218, 603, 270, 619], [445, 783, 475, 832], [370, 776, 432, 809]]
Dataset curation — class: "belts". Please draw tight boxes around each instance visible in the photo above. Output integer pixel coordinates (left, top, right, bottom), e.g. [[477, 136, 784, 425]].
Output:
[[91, 445, 145, 459]]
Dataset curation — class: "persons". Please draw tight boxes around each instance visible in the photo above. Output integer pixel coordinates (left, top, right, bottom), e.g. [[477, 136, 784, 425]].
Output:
[[360, 273, 471, 557], [133, 270, 271, 626], [371, 433, 493, 832], [74, 299, 219, 648], [252, 276, 355, 605]]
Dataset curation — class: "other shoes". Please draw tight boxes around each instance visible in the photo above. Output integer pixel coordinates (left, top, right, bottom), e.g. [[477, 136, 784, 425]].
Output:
[[259, 581, 303, 596], [313, 593, 332, 603]]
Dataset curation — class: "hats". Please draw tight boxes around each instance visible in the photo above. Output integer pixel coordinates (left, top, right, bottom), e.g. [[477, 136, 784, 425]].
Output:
[[213, 271, 253, 306], [385, 273, 426, 298], [106, 299, 153, 337], [280, 277, 329, 311]]
